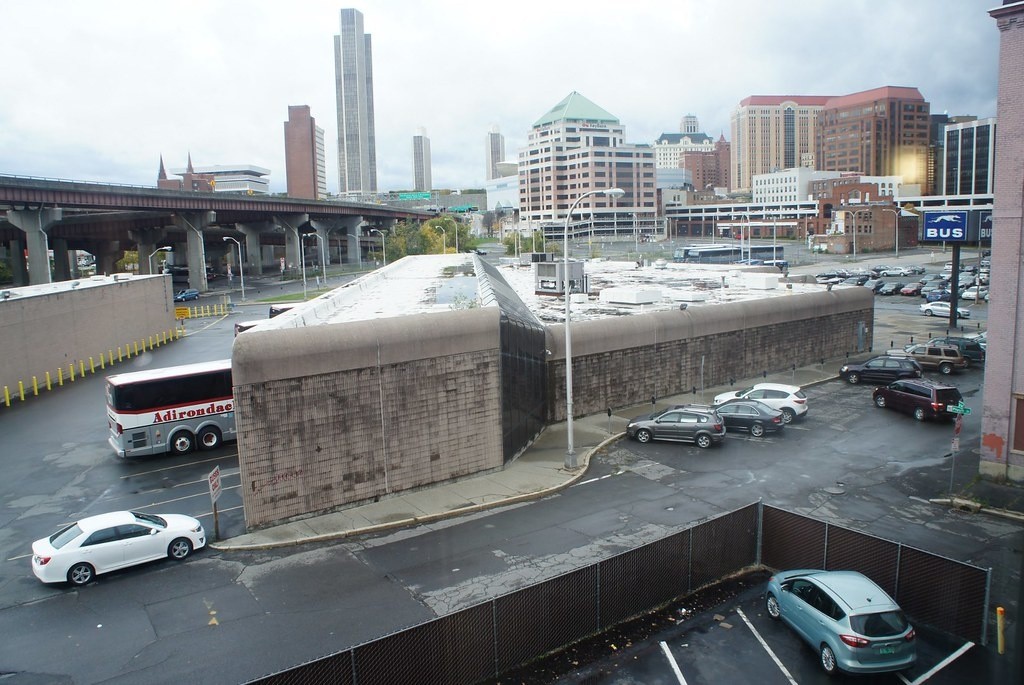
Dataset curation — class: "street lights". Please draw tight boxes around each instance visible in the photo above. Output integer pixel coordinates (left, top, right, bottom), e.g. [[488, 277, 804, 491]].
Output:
[[347, 233, 359, 265], [831, 209, 869, 257], [499, 217, 508, 243], [882, 208, 912, 257], [564, 187, 626, 455], [301, 233, 308, 297], [149, 246, 172, 274], [444, 218, 459, 253], [370, 228, 385, 267], [307, 233, 327, 285], [628, 212, 638, 253], [435, 226, 445, 253]]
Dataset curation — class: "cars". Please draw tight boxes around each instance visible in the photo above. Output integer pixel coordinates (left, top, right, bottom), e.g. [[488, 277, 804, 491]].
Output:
[[31, 510, 206, 587], [712, 398, 785, 437], [814, 249, 991, 320], [174, 288, 199, 302], [466, 249, 487, 255], [766, 568, 918, 676]]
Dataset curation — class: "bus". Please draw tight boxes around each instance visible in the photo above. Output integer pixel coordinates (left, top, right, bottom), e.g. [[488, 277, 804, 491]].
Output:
[[158, 265, 216, 284], [105, 358, 237, 457], [673, 244, 789, 278], [269, 302, 304, 318], [234, 319, 271, 338]]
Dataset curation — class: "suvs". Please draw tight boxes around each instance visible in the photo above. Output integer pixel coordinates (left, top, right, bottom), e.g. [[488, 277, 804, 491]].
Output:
[[872, 377, 965, 423], [77, 260, 91, 268], [714, 383, 809, 425], [838, 355, 923, 385], [625, 403, 727, 449], [903, 336, 983, 368], [884, 343, 966, 375]]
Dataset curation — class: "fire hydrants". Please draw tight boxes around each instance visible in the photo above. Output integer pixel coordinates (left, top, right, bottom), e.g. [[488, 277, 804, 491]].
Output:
[[223, 236, 245, 301]]
